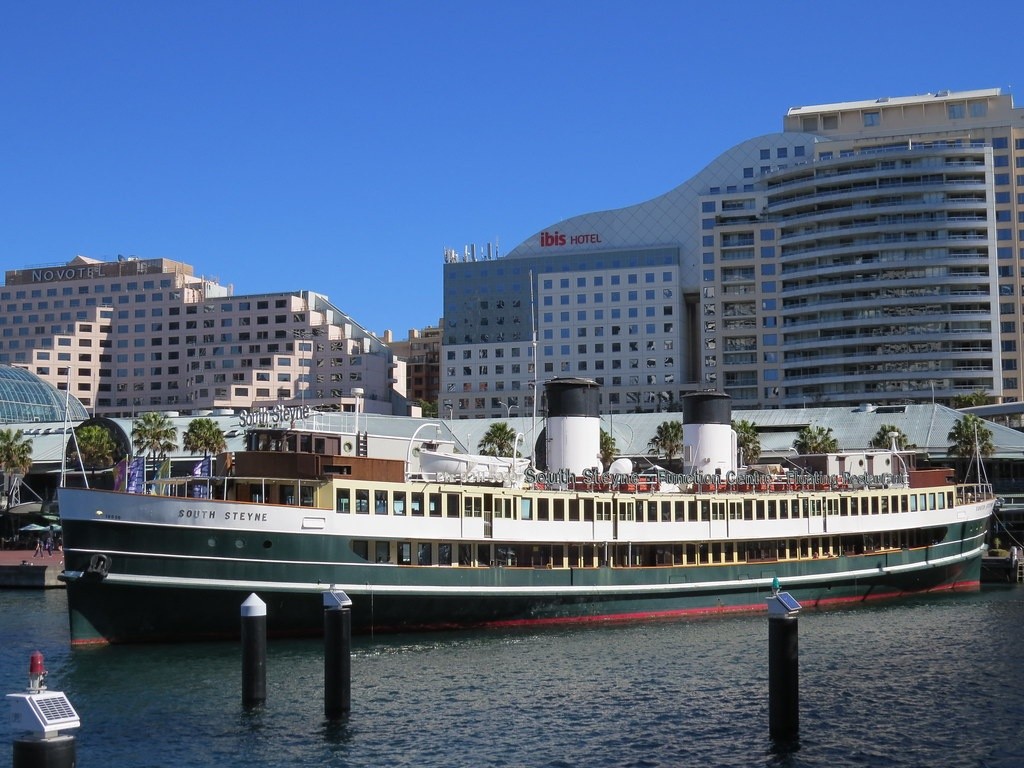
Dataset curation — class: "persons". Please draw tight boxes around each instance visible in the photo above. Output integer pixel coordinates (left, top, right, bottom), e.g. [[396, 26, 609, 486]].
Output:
[[46, 537, 53, 558], [32, 538, 44, 559], [58, 534, 63, 553]]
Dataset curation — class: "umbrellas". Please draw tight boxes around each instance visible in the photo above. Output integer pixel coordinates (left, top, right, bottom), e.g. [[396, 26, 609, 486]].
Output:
[[46, 523, 62, 531], [18, 523, 48, 535]]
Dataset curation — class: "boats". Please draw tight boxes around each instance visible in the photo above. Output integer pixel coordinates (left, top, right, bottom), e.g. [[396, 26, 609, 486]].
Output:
[[55, 268, 1000, 651], [418, 447, 530, 486]]
